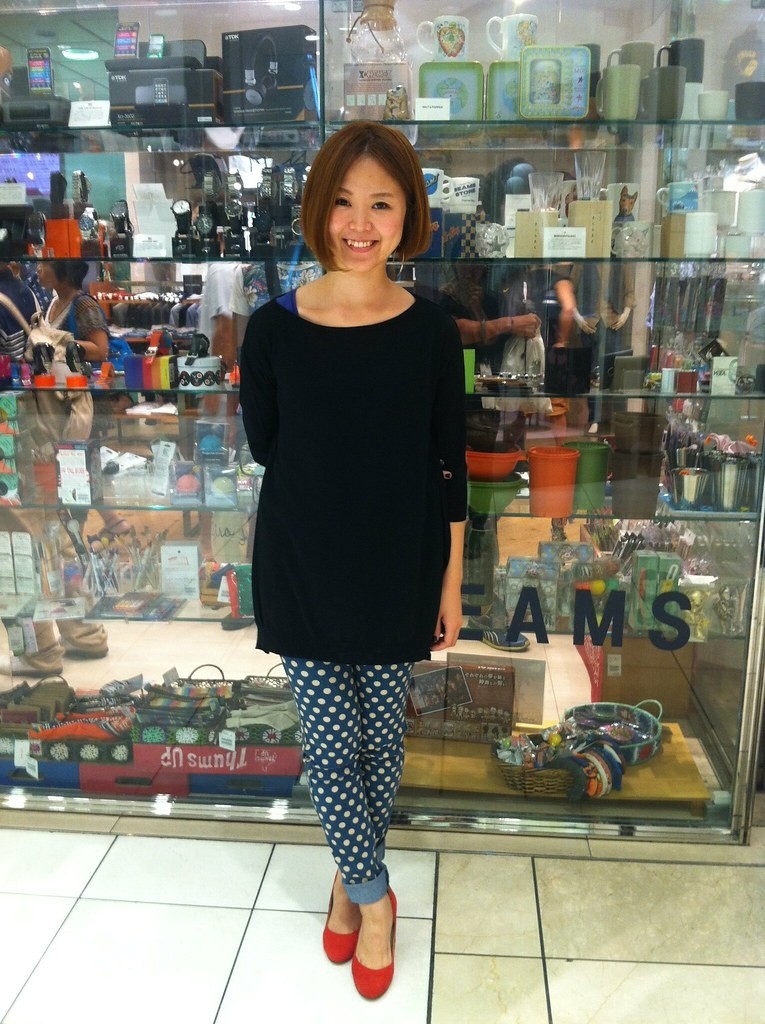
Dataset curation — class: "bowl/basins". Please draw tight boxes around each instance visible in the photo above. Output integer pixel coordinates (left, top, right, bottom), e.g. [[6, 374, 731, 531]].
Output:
[[467, 410, 527, 452], [466, 472, 526, 515], [466, 442, 526, 479]]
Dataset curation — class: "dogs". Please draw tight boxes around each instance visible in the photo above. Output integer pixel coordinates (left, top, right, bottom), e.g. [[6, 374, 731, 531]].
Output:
[[615, 185, 639, 222]]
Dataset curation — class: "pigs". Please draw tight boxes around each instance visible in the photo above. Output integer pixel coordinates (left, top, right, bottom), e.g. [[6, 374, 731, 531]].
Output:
[[477, 223, 509, 256], [615, 226, 650, 259]]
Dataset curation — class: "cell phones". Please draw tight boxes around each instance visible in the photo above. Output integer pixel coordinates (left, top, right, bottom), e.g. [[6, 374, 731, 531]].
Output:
[[113, 21, 141, 59], [26, 46, 54, 95], [147, 33, 165, 58], [154, 78, 169, 105]]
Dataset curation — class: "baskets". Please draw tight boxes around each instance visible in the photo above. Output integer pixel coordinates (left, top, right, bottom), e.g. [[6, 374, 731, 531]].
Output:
[[490, 746, 574, 797]]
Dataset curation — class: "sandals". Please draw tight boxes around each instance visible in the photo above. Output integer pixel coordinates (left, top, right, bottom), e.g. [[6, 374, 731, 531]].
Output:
[[89, 519, 131, 546]]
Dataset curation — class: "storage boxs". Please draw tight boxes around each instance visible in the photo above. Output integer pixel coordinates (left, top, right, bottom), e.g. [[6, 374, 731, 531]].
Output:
[[601, 637, 695, 718], [0, 428, 33, 475], [0, 465, 36, 507], [515, 212, 558, 256], [19, 455, 56, 504], [488, 61, 518, 121], [108, 69, 223, 127], [0, 390, 35, 435], [3, 94, 72, 124], [519, 47, 591, 120], [417, 208, 443, 258], [421, 60, 484, 117], [503, 194, 529, 229], [567, 198, 613, 257], [29, 216, 82, 257], [0, 729, 308, 797], [124, 352, 176, 388], [445, 213, 490, 258], [343, 62, 415, 120], [220, 25, 318, 123], [54, 439, 104, 506], [490, 541, 684, 641]]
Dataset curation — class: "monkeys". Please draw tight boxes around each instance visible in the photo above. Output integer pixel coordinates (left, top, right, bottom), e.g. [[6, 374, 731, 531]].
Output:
[[383, 84, 411, 121]]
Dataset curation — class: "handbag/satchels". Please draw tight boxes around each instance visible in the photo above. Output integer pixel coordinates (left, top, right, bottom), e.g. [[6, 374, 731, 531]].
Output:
[[492, 326, 552, 416], [70, 293, 135, 371]]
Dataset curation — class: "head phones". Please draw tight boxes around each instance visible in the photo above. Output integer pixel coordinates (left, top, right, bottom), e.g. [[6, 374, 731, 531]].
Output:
[[245, 37, 278, 105]]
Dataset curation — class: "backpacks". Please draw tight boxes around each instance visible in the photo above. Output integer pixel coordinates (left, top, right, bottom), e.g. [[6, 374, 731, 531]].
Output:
[[0, 290, 94, 448]]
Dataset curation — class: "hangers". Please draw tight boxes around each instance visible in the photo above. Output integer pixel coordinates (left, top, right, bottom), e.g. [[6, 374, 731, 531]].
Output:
[[119, 291, 204, 304]]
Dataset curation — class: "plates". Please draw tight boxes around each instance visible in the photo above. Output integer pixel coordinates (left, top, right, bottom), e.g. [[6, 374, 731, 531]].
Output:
[[417, 44, 590, 124]]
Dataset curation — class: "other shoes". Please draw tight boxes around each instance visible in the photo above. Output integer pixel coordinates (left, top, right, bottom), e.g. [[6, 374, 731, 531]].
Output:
[[2, 656, 63, 677], [323, 869, 362, 963], [61, 643, 109, 661], [350, 889, 397, 999]]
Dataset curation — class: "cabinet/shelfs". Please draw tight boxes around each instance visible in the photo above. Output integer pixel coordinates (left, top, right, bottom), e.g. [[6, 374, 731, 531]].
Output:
[[0, 0, 325, 846], [324, 0, 765, 846]]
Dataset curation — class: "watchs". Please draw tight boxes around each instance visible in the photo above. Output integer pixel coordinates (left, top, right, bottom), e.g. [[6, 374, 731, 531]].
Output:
[[170, 166, 311, 253], [27, 170, 129, 244]]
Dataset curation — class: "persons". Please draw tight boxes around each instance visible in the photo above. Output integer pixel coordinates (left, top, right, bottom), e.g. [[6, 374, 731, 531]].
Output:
[[201, 261, 320, 631], [574, 262, 638, 433], [435, 157, 539, 653], [0, 259, 130, 676], [506, 261, 574, 347], [236, 121, 466, 1000]]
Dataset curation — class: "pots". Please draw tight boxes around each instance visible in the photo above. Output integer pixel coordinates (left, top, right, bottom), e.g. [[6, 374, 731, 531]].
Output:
[[560, 699, 663, 766]]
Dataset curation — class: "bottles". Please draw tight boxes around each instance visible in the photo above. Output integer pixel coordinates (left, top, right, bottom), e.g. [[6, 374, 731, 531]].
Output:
[[351, 0, 404, 62]]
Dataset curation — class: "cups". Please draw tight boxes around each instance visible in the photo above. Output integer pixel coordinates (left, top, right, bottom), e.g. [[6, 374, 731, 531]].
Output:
[[422, 167, 480, 213], [580, 37, 765, 122], [485, 13, 539, 60], [415, 14, 469, 61], [528, 446, 581, 518], [563, 441, 611, 510], [612, 410, 668, 452], [656, 178, 765, 259], [611, 221, 654, 258], [612, 450, 664, 517], [527, 171, 564, 211], [574, 150, 606, 200], [667, 446, 762, 512]]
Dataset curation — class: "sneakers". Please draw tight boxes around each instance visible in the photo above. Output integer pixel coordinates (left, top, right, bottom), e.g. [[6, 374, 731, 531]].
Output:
[[466, 621, 532, 651]]
